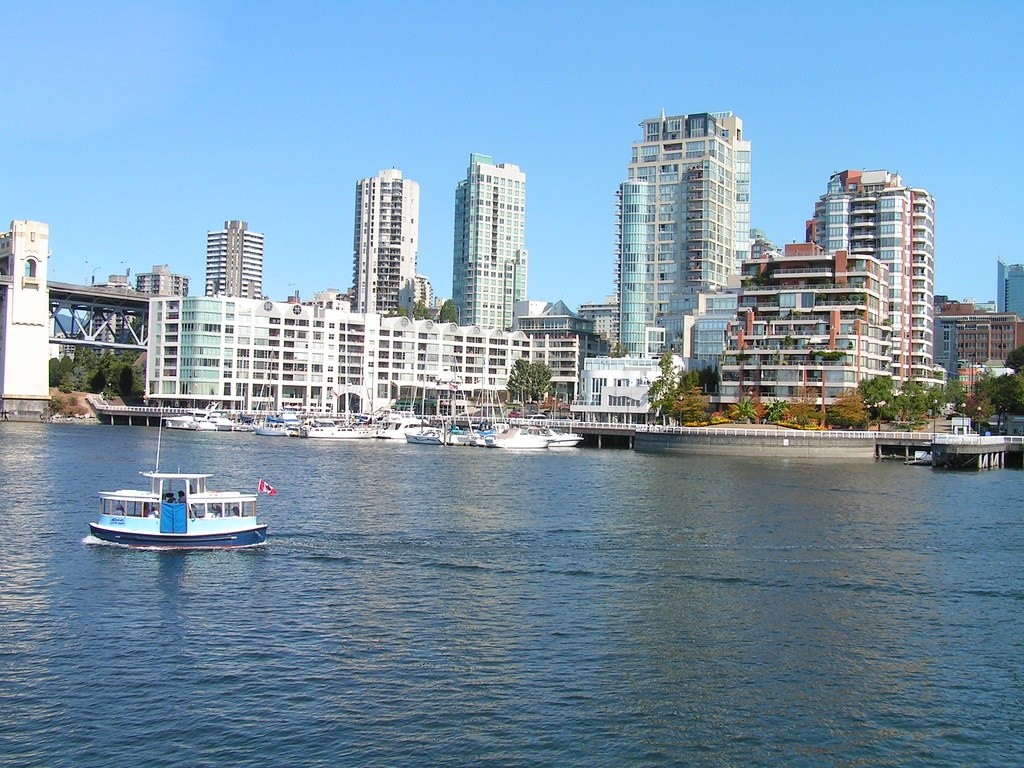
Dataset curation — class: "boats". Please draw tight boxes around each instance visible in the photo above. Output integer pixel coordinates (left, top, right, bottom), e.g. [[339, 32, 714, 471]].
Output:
[[87, 403, 269, 547]]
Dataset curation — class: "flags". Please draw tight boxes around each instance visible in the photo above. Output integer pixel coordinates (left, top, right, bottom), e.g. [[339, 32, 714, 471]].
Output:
[[258, 478, 277, 496]]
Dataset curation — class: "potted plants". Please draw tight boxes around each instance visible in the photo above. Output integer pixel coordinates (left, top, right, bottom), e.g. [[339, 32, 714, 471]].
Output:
[[847, 263, 855, 272], [784, 335, 794, 350], [816, 293, 867, 305], [825, 279, 865, 288]]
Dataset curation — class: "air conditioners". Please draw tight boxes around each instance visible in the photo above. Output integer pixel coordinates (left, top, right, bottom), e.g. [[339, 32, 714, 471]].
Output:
[[688, 165, 695, 280]]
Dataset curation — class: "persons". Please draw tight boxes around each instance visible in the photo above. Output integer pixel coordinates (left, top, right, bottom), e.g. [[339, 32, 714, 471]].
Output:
[[647, 417, 656, 431], [117, 484, 239, 519], [481, 422, 485, 432], [614, 416, 618, 428]]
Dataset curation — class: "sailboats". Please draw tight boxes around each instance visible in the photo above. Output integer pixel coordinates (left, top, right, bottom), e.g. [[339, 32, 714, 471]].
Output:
[[158, 350, 584, 451]]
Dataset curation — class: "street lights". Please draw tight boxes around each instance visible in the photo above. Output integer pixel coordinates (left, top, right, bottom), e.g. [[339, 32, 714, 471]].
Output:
[[749, 391, 754, 419], [932, 398, 939, 434], [875, 400, 887, 431], [678, 396, 683, 427], [961, 402, 966, 435], [976, 406, 981, 435]]
